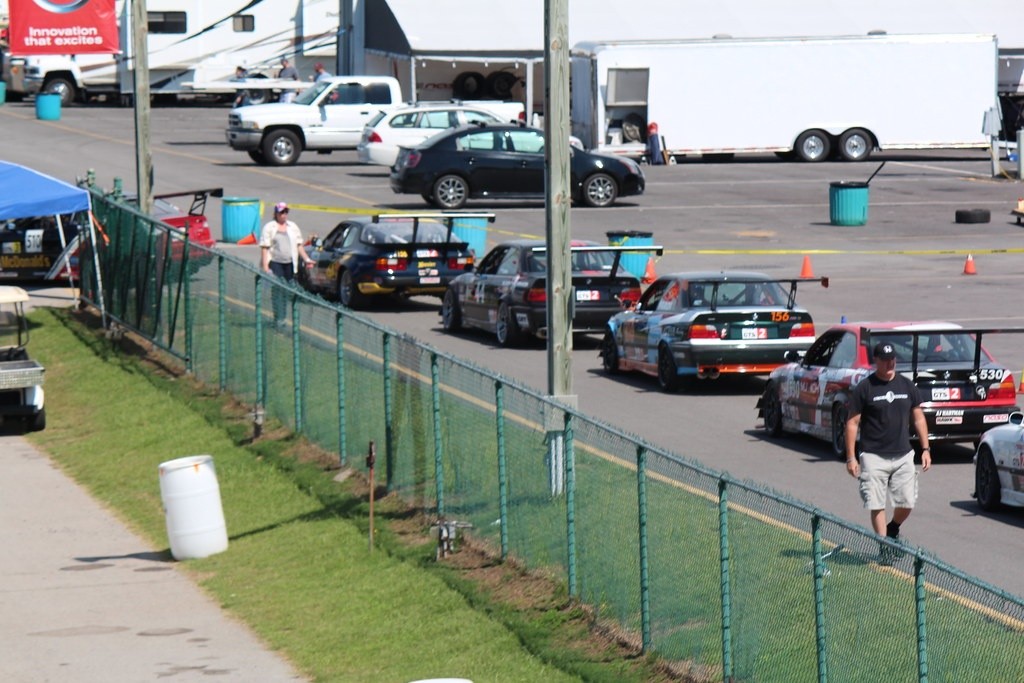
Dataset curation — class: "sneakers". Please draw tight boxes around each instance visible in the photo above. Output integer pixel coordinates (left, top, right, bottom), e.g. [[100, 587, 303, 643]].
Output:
[[887, 525, 904, 558], [876, 546, 892, 567]]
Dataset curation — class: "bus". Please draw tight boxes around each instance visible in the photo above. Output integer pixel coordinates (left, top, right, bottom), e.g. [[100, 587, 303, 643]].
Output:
[[2, 0, 339, 108]]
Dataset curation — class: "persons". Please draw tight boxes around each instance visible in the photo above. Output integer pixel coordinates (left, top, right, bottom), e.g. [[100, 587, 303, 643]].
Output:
[[310, 62, 331, 102], [278, 58, 300, 103], [235, 67, 250, 107], [261, 204, 316, 327], [844, 342, 931, 564]]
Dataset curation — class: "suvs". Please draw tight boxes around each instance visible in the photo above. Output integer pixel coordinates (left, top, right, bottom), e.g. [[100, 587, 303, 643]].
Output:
[[356, 101, 582, 173]]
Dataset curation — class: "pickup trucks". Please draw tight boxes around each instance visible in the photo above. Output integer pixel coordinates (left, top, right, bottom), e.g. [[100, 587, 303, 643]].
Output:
[[224, 74, 526, 166]]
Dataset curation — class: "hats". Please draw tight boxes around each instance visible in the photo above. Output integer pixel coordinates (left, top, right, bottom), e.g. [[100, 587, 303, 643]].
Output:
[[275, 203, 291, 213], [874, 343, 895, 360]]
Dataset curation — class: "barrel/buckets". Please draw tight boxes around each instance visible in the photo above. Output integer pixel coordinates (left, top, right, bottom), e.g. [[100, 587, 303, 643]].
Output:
[[606, 231, 654, 283], [0, 82, 6, 105], [222, 197, 260, 243], [157, 455, 230, 559], [35, 92, 61, 120], [828, 181, 869, 226], [442, 210, 489, 259]]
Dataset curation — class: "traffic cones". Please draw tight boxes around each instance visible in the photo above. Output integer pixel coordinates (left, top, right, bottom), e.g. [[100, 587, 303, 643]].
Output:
[[799, 255, 815, 278], [961, 254, 979, 275], [640, 259, 657, 284]]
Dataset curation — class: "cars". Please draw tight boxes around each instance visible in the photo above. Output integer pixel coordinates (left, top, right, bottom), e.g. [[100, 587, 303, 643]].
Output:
[[439, 238, 664, 349], [754, 321, 1021, 462], [972, 411, 1024, 514], [598, 270, 830, 393], [387, 119, 644, 210], [0, 188, 224, 288], [298, 211, 496, 311]]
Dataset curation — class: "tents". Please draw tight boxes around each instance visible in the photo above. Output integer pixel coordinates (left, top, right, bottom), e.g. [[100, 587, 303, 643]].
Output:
[[0, 160, 106, 327]]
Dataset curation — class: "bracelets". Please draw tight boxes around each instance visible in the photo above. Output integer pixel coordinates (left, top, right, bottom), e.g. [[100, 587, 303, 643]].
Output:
[[922, 447, 930, 450], [847, 458, 855, 462]]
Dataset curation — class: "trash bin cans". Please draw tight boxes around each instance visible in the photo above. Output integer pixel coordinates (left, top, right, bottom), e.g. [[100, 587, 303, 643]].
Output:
[[442, 212, 488, 257], [157, 456, 227, 559], [222, 198, 261, 243], [0, 81, 7, 105], [828, 181, 870, 225], [36, 93, 62, 121], [607, 231, 655, 280]]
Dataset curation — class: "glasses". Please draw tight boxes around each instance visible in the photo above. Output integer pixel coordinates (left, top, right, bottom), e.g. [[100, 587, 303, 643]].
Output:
[[280, 210, 289, 214]]
